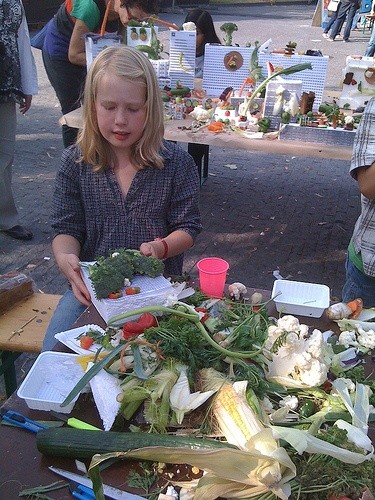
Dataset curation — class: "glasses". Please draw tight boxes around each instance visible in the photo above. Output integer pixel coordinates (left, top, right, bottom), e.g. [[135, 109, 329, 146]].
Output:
[[126, 6, 149, 22]]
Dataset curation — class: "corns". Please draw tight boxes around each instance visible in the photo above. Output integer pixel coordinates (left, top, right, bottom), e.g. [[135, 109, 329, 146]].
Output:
[[198, 368, 287, 483]]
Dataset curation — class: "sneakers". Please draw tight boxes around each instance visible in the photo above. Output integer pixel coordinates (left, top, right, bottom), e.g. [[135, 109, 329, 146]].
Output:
[[335, 35, 340, 39], [326, 38, 334, 42], [343, 40, 349, 43], [322, 33, 329, 39]]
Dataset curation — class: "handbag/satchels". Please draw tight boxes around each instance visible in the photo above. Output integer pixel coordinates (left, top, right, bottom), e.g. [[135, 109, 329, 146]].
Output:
[[30, 22, 49, 49]]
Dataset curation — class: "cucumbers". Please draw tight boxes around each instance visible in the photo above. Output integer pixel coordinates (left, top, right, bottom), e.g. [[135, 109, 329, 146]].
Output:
[[37, 428, 238, 453]]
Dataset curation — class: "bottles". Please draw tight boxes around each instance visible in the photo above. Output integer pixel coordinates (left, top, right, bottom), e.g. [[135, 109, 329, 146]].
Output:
[[171, 98, 188, 121]]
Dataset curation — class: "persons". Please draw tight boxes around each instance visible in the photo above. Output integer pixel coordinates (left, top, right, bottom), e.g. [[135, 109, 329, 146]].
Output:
[[363, 4, 375, 57], [0, 0, 38, 240], [184, 9, 223, 78], [327, 0, 361, 43], [342, 95, 375, 309], [41, 45, 205, 355], [42, 0, 155, 150], [322, 0, 344, 38]]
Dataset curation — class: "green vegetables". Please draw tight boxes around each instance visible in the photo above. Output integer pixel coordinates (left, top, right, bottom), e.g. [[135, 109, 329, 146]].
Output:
[[118, 285, 375, 500], [89, 249, 164, 301]]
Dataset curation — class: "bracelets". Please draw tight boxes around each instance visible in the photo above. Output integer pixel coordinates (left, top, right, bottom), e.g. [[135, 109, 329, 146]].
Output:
[[154, 238, 168, 261]]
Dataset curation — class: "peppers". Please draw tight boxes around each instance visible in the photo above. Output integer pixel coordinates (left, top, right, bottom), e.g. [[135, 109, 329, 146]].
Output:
[[122, 312, 158, 341]]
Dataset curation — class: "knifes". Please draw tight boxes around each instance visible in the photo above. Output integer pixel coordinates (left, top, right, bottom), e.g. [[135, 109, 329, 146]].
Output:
[[48, 465, 149, 500]]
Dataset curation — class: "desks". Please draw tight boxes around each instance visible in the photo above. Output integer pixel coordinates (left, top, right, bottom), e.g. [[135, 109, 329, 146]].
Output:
[[0, 274, 375, 500], [58, 78, 356, 188]]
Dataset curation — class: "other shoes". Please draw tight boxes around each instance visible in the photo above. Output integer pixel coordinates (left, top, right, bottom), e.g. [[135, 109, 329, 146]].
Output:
[[7, 224, 32, 241]]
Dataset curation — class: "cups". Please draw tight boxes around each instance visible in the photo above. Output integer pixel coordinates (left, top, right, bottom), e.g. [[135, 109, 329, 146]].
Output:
[[196, 257, 229, 299]]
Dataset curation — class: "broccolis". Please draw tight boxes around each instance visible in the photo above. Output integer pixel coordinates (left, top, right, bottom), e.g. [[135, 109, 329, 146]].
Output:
[[253, 315, 334, 385]]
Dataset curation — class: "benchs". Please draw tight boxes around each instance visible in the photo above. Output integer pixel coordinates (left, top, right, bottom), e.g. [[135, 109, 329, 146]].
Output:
[[0, 293, 64, 399]]
